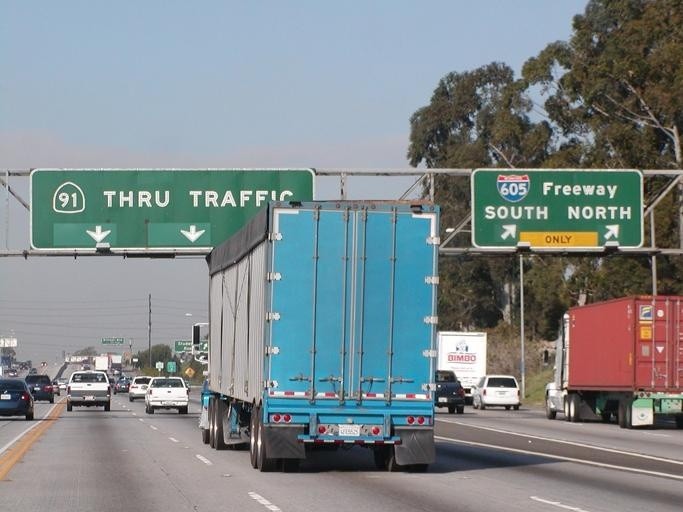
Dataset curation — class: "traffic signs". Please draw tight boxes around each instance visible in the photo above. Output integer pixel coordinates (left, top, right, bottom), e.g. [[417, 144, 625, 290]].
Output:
[[101, 337, 125, 346], [29, 167, 316, 250], [470, 166, 643, 252], [175, 340, 208, 353]]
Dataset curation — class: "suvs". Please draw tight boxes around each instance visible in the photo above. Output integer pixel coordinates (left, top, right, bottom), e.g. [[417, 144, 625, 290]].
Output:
[[143, 376, 189, 415], [25, 375, 54, 403], [471, 374, 520, 409], [65, 370, 110, 412], [128, 376, 151, 402]]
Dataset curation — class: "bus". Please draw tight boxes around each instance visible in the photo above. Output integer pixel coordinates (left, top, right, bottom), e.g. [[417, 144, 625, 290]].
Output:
[[82, 359, 90, 367]]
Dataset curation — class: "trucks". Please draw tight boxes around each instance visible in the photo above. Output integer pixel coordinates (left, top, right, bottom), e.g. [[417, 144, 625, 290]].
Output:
[[435, 330, 487, 407], [93, 356, 111, 373], [543, 295, 682, 429], [191, 200, 441, 472]]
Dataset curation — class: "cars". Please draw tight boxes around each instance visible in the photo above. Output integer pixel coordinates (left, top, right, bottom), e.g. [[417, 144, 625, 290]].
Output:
[[83, 366, 89, 371], [434, 370, 466, 413], [58, 383, 66, 390], [50, 381, 60, 397], [4, 359, 48, 378], [0, 379, 34, 422], [109, 373, 129, 394]]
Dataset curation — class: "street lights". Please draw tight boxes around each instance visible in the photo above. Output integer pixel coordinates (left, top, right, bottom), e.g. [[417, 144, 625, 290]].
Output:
[[9, 328, 15, 357], [128, 338, 134, 364], [185, 313, 207, 320], [445, 227, 527, 402]]
